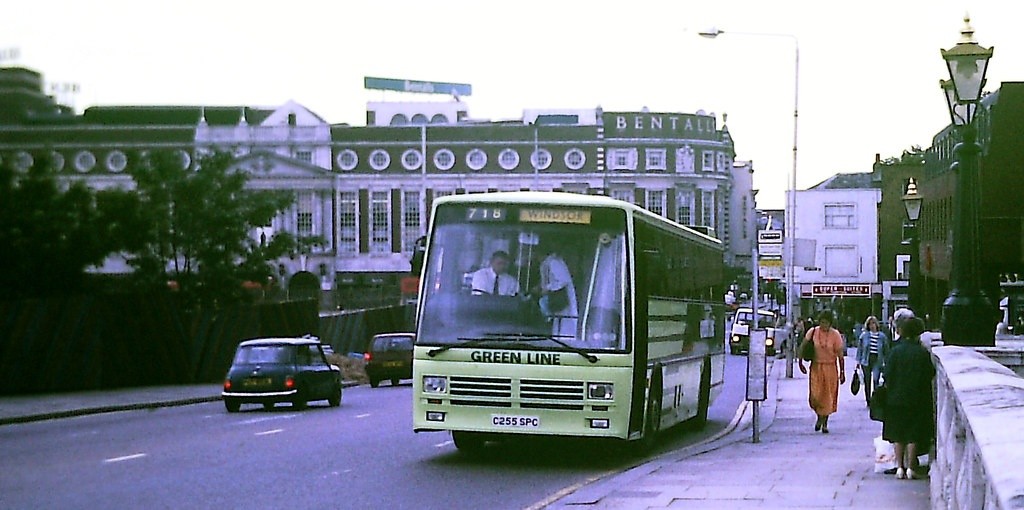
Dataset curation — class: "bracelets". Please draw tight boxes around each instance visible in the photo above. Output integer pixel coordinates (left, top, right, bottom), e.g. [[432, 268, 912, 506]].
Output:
[[840, 370, 844, 373]]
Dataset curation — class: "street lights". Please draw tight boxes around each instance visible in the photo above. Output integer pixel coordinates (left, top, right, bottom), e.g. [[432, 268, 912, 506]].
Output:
[[901, 178, 926, 319], [697, 27, 801, 378], [939, 12, 996, 347]]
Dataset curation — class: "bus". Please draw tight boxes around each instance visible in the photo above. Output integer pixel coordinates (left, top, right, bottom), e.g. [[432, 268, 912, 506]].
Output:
[[409, 190, 728, 454]]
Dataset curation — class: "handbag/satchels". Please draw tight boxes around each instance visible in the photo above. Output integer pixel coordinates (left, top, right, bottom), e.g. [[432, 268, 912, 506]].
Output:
[[547, 286, 570, 313], [804, 328, 816, 361], [870, 382, 886, 421], [851, 367, 860, 395]]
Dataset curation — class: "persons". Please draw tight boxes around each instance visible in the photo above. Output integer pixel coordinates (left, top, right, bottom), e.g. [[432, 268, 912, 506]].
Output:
[[798, 310, 846, 433], [530, 244, 579, 341], [855, 309, 926, 473], [856, 315, 888, 406], [472, 251, 520, 296], [792, 313, 814, 362], [882, 317, 936, 480]]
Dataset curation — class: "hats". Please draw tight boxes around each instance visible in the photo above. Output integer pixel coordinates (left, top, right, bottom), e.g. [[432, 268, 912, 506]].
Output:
[[902, 317, 923, 335]]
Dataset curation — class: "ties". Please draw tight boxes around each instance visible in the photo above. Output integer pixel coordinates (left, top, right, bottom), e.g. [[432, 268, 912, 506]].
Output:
[[493, 275, 499, 294]]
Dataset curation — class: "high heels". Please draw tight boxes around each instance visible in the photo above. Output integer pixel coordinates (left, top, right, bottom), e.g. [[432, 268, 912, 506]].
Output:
[[906, 468, 918, 480], [896, 468, 905, 479]]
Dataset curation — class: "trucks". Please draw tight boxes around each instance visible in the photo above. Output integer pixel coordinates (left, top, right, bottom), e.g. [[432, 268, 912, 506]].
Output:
[[729, 306, 776, 355]]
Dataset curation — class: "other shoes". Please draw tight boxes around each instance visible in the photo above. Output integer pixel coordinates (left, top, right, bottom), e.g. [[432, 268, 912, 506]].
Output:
[[822, 428, 828, 433], [814, 425, 821, 431], [884, 468, 896, 474]]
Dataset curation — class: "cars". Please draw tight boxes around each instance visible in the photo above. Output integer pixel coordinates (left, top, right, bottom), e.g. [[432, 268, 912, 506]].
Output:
[[220, 338, 342, 412], [363, 332, 416, 387]]
